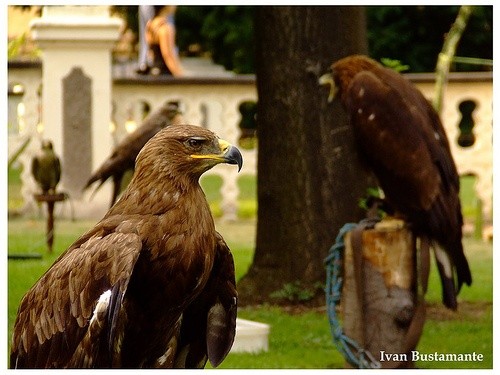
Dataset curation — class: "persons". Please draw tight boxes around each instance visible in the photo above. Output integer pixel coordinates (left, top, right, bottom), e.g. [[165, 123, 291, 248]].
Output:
[[143, 5, 186, 78]]
